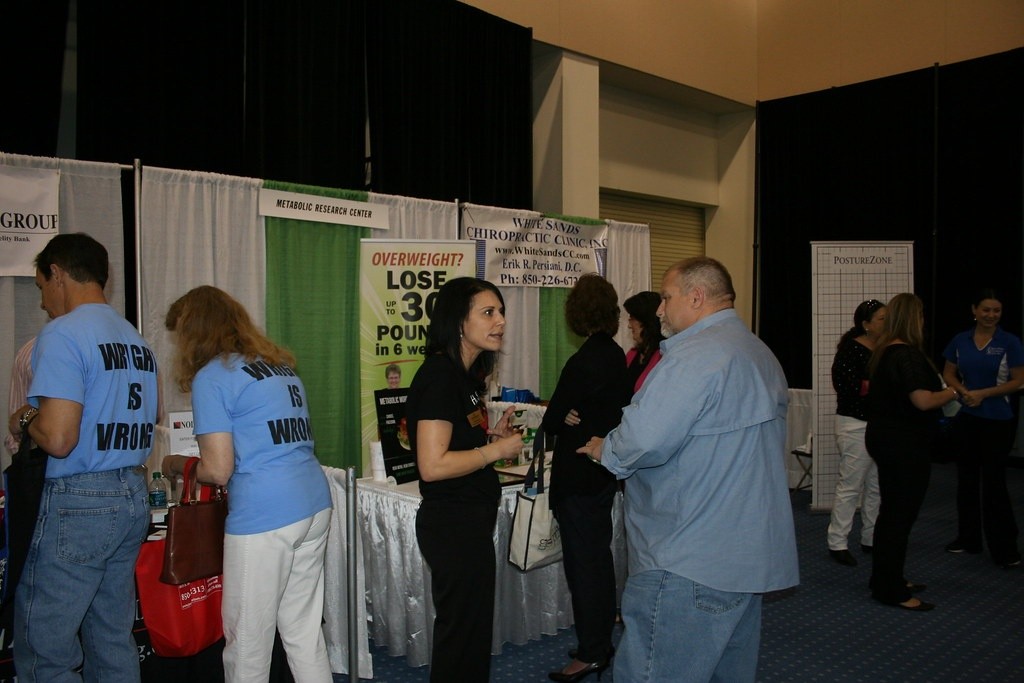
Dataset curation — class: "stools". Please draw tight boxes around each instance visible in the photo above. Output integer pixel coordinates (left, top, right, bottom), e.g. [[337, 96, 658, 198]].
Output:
[[791, 449, 812, 498]]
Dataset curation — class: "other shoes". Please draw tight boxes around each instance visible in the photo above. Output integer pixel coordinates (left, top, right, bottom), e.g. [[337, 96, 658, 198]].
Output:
[[860, 544, 873, 553], [871, 583, 925, 600], [883, 596, 933, 612], [944, 537, 975, 553], [999, 556, 1019, 568]]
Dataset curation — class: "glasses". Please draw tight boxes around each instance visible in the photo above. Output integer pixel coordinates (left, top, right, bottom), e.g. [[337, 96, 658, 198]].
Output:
[[862, 300, 878, 319]]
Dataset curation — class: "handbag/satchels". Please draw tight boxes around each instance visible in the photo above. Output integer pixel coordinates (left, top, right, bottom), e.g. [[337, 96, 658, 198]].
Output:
[[160, 461, 228, 585], [508, 424, 563, 572], [135, 457, 224, 657], [0, 413, 49, 601]]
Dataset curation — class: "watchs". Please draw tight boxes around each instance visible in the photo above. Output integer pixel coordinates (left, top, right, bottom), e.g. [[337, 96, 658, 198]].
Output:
[[20, 408, 36, 427]]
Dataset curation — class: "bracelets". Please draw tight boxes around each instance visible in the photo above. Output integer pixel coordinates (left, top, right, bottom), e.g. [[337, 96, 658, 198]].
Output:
[[947, 387, 956, 400], [474, 447, 487, 469]]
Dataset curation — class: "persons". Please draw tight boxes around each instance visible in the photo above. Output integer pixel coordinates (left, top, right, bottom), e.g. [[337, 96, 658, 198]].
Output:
[[865, 293, 959, 611], [381, 364, 401, 390], [576, 255, 802, 683], [622, 291, 668, 393], [404, 276, 525, 683], [7, 231, 163, 683], [942, 288, 1024, 568], [827, 299, 887, 567], [160, 285, 333, 683], [541, 272, 634, 683]]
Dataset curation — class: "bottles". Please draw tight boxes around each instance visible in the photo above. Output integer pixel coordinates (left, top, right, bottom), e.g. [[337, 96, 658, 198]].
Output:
[[148, 471, 168, 509], [171, 472, 186, 503], [807, 433, 812, 453]]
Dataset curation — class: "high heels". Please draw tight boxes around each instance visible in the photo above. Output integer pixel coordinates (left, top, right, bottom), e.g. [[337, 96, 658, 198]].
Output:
[[548, 659, 610, 683], [568, 645, 616, 667], [829, 549, 857, 566]]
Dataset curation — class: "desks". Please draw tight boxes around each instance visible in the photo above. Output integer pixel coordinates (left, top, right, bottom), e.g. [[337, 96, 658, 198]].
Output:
[[355, 456, 576, 665]]
